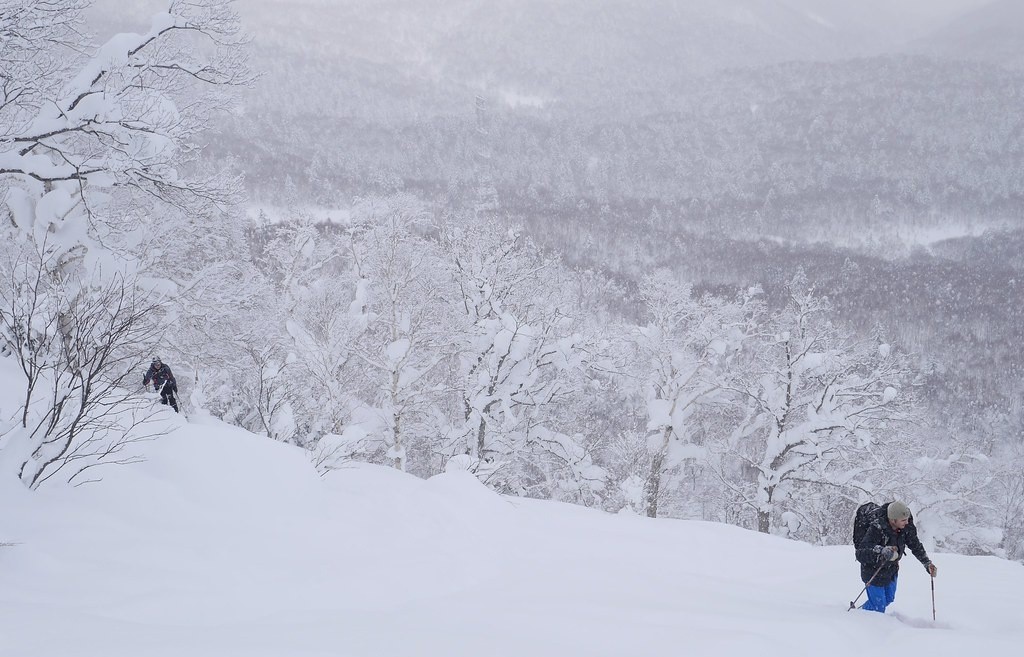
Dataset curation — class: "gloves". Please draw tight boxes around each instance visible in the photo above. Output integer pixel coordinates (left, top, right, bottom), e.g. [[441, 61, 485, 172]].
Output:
[[173, 387, 177, 392], [881, 546, 894, 560], [926, 564, 937, 577], [143, 379, 150, 384]]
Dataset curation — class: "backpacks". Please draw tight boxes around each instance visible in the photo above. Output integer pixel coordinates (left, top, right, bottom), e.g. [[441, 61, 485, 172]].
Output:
[[853, 501, 889, 549]]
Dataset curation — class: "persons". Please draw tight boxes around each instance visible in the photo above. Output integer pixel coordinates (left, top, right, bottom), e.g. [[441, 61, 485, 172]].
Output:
[[855, 502, 937, 613], [142, 355, 179, 413]]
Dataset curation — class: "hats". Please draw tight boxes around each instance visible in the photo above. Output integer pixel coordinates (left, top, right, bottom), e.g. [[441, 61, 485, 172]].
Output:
[[887, 501, 911, 518], [151, 356, 161, 363]]
